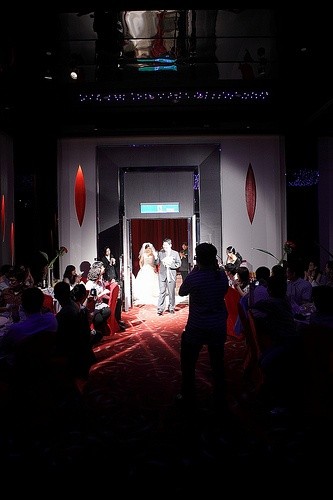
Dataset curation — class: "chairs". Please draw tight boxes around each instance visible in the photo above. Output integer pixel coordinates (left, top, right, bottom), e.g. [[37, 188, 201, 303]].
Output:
[[224, 287, 262, 372], [106, 286, 120, 336]]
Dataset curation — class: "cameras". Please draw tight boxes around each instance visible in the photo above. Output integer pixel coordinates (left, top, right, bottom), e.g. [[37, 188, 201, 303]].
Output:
[[91, 289, 97, 296]]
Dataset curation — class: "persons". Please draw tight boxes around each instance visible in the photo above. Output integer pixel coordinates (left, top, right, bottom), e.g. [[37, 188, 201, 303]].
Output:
[[0, 238, 333, 422]]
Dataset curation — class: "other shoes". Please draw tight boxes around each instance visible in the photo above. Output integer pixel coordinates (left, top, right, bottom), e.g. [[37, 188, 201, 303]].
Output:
[[157, 311, 162, 315], [170, 310, 175, 314]]
